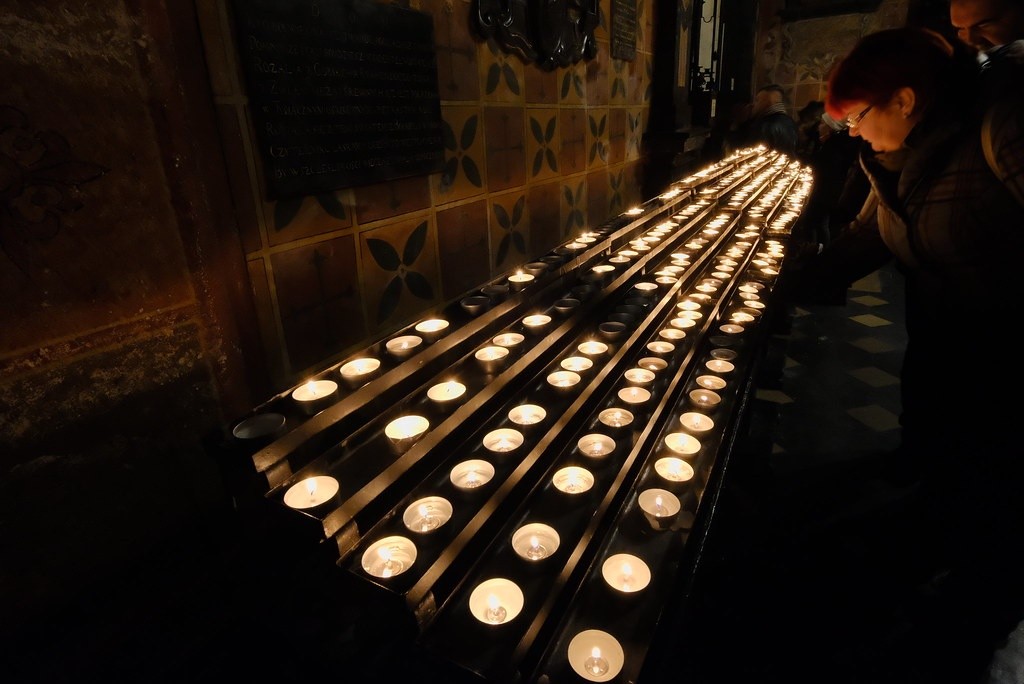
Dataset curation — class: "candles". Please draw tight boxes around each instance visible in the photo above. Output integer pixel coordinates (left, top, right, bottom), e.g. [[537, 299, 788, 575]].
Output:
[[231, 142, 814, 684]]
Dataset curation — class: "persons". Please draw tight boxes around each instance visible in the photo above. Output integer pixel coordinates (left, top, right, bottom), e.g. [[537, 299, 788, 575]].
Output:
[[721, 85, 875, 253], [795, 23, 1023, 567], [943, 0, 1024, 54]]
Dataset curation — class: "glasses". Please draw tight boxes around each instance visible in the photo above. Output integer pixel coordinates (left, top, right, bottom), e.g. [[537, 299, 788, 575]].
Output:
[[845, 104, 875, 129]]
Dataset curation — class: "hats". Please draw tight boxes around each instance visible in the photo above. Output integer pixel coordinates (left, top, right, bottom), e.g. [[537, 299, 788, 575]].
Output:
[[822, 112, 844, 132]]
